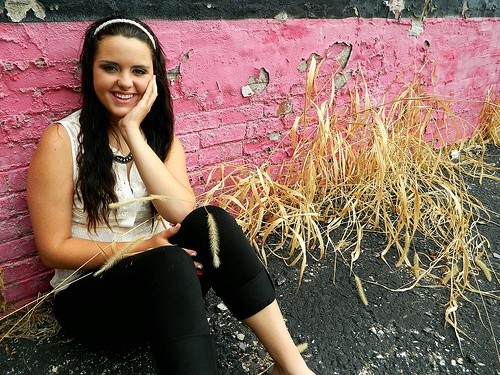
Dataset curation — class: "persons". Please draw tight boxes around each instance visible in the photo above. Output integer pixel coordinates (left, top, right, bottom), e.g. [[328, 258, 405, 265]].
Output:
[[25, 16, 321, 375]]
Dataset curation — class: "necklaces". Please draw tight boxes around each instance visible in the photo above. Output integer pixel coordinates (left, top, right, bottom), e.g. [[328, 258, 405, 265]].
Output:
[[107, 146, 135, 164]]
